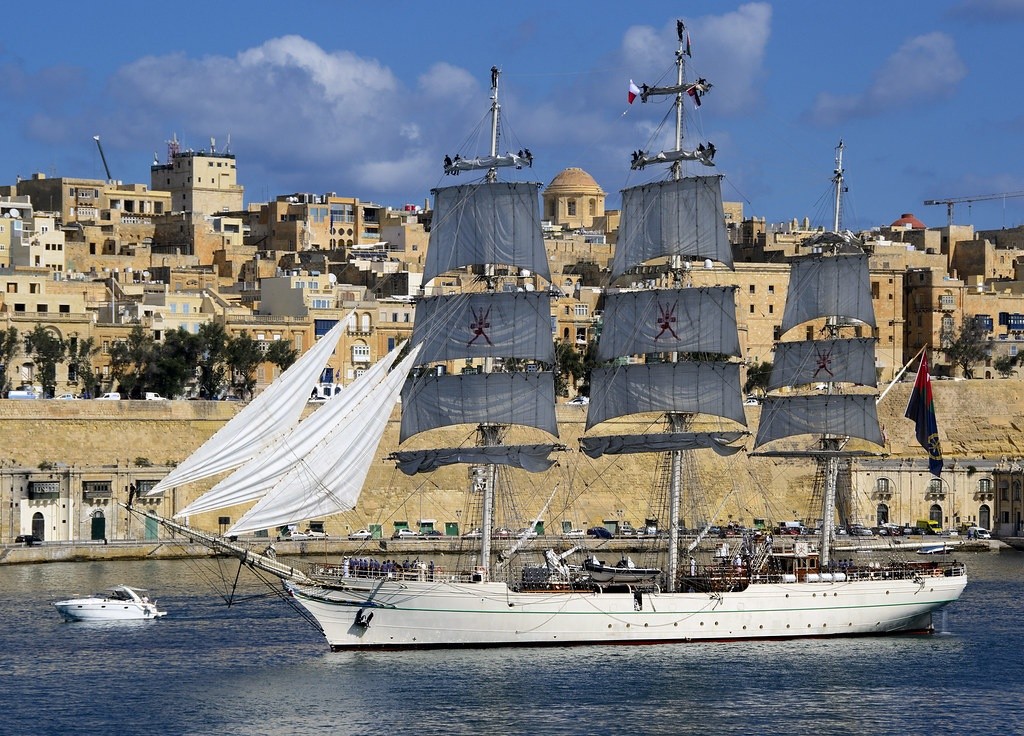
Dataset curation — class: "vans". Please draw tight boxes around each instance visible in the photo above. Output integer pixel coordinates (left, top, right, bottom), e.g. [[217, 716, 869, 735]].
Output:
[[95, 393, 120, 400], [146, 392, 166, 400]]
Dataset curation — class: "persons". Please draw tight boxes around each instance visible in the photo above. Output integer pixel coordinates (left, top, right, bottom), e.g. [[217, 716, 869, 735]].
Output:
[[707, 141, 716, 161], [689, 555, 695, 576], [637, 83, 650, 104], [443, 154, 452, 175], [452, 154, 460, 175], [698, 77, 706, 96], [129, 483, 136, 506], [491, 65, 497, 87], [638, 149, 645, 171], [677, 20, 685, 41], [516, 149, 523, 170], [342, 556, 434, 575], [524, 147, 533, 168], [696, 143, 705, 151], [630, 151, 638, 170], [829, 559, 854, 568]]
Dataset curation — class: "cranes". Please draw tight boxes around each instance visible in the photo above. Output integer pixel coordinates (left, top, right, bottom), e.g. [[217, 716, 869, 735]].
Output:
[[924, 191, 1024, 227]]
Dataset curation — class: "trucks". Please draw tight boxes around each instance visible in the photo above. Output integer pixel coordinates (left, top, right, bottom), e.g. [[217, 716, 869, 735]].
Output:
[[917, 520, 942, 534]]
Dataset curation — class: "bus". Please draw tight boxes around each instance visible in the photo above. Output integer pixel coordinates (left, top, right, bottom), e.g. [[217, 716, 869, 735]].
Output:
[[8, 391, 36, 399]]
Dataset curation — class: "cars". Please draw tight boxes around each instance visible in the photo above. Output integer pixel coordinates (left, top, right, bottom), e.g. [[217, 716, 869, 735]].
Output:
[[462, 527, 538, 539], [700, 526, 926, 535], [563, 525, 644, 535]]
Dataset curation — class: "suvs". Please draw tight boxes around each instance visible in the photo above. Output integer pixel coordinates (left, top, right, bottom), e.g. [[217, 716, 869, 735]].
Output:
[[220, 396, 241, 401], [15, 535, 42, 545], [974, 529, 991, 539], [394, 529, 441, 540], [284, 529, 330, 541]]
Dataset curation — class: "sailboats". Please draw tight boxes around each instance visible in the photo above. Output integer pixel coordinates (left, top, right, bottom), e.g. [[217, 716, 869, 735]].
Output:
[[116, 20, 968, 651]]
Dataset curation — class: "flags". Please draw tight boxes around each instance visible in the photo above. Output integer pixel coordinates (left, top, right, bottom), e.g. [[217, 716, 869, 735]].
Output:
[[905, 348, 944, 478], [687, 86, 702, 110], [627, 79, 640, 104], [687, 31, 691, 58]]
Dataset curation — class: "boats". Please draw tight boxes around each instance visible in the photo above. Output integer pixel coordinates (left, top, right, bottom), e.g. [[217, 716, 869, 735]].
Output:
[[582, 555, 661, 582], [54, 584, 167, 622]]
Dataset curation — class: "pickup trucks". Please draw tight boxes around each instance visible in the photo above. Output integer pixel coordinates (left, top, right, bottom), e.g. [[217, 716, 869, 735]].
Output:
[[53, 394, 83, 400], [348, 530, 371, 540]]
